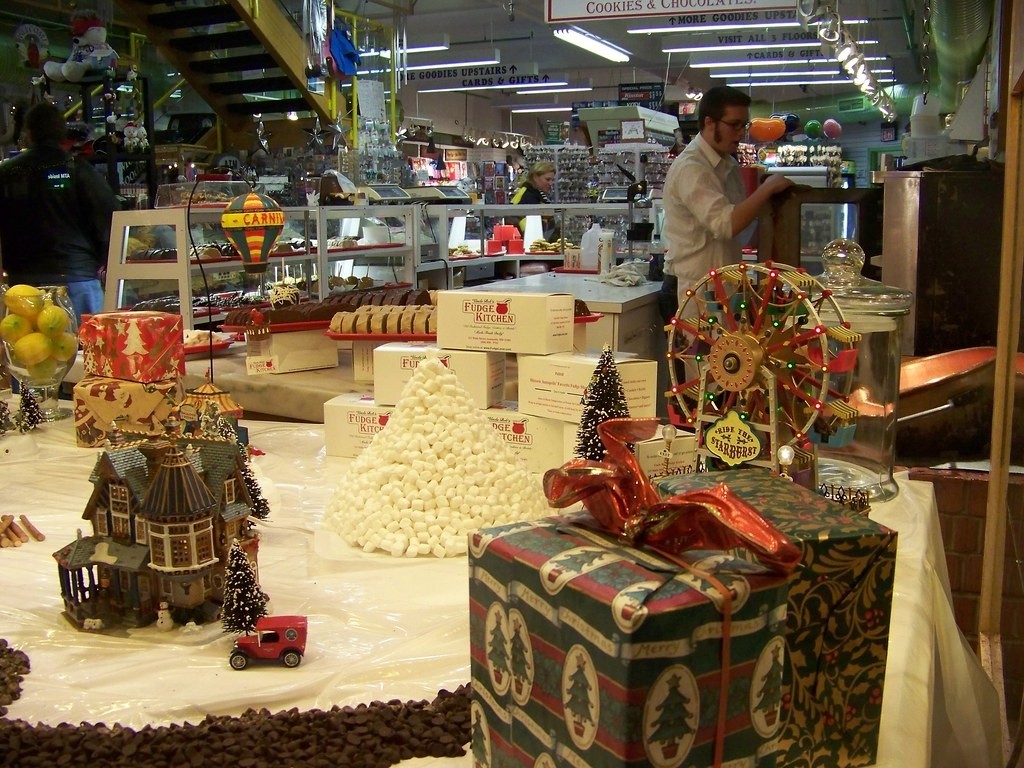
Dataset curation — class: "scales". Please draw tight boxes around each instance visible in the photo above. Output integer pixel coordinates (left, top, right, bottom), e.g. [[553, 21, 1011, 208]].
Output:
[[602, 187, 628, 203], [403, 186, 470, 204], [356, 184, 412, 204]]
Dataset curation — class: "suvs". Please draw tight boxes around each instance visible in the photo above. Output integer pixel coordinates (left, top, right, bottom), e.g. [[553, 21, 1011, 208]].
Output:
[[230, 615, 309, 669]]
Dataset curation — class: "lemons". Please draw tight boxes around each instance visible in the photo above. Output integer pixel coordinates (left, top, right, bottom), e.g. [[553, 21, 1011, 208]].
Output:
[[0, 284, 77, 383]]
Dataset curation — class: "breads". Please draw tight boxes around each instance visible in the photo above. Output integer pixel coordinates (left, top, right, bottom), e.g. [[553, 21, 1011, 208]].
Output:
[[449, 244, 472, 256], [530, 238, 578, 251], [130, 237, 438, 347]]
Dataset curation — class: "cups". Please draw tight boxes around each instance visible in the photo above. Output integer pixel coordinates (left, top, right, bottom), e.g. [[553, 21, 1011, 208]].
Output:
[[563, 249, 579, 269]]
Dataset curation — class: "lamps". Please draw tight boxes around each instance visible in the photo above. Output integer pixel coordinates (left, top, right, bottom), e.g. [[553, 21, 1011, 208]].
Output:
[[510, 134, 520, 149], [481, 131, 490, 146], [462, 128, 469, 142], [501, 134, 509, 149], [520, 137, 528, 151], [524, 138, 533, 150], [469, 129, 477, 143], [477, 129, 482, 146], [492, 133, 502, 147], [115, 19, 900, 123]]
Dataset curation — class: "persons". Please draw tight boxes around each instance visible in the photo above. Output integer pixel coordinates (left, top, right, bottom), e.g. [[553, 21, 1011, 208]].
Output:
[[0, 101, 123, 401], [658, 86, 796, 472], [504, 162, 561, 244]]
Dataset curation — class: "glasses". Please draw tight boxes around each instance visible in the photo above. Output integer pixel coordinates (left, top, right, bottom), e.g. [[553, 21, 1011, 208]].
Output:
[[712, 116, 752, 131]]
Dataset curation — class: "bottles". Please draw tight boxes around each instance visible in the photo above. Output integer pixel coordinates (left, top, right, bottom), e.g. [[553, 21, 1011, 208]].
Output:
[[805, 240, 915, 503], [579, 219, 603, 271]]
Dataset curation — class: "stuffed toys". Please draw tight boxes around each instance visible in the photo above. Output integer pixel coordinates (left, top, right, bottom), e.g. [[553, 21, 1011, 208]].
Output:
[[43, 17, 121, 84], [102, 87, 152, 155]]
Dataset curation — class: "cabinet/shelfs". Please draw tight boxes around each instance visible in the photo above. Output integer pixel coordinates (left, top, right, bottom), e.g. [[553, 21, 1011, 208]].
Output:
[[102, 144, 1006, 357]]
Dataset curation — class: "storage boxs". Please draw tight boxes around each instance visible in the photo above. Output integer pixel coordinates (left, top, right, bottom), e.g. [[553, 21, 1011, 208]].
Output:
[[485, 191, 495, 204], [620, 118, 646, 144], [80, 309, 187, 385], [517, 351, 658, 424], [495, 161, 508, 175], [487, 240, 502, 254], [247, 328, 339, 376], [651, 467, 900, 768], [351, 339, 388, 382], [323, 393, 394, 459], [493, 176, 508, 190], [482, 161, 496, 177], [484, 177, 495, 191], [483, 400, 563, 487], [436, 290, 575, 355], [74, 375, 186, 448], [563, 421, 696, 484], [467, 511, 787, 768], [506, 239, 523, 254], [493, 224, 515, 246], [152, 181, 267, 209], [245, 354, 279, 376], [330, 193, 370, 205], [545, 120, 571, 145], [373, 342, 507, 411], [494, 191, 508, 204]]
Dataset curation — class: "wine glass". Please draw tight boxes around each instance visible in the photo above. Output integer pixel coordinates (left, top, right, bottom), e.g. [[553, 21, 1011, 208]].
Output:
[[0, 285, 78, 421]]
